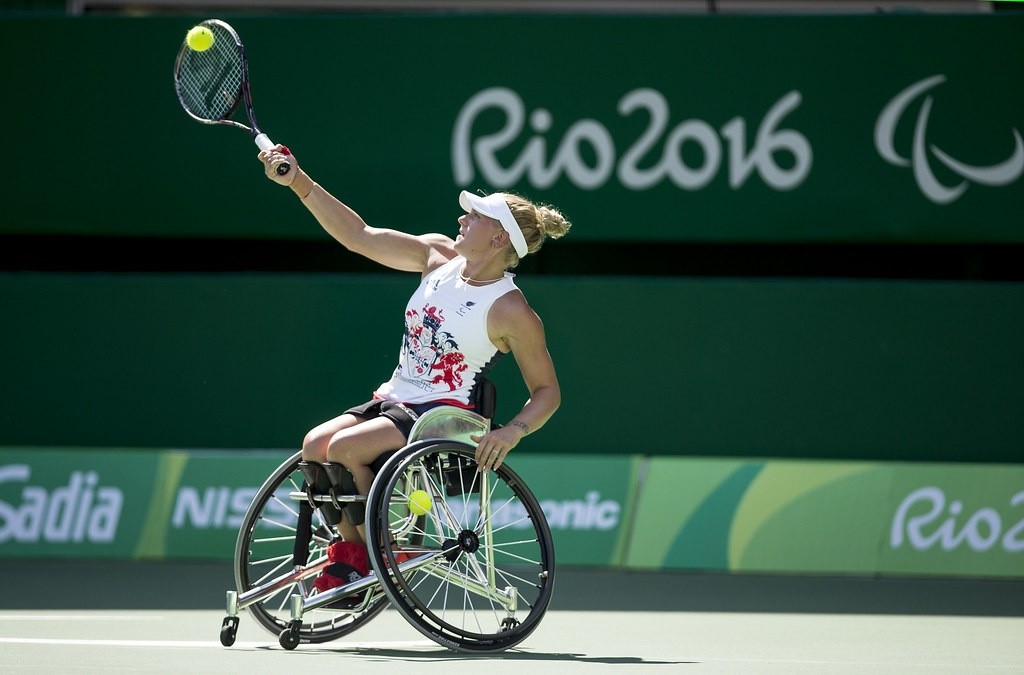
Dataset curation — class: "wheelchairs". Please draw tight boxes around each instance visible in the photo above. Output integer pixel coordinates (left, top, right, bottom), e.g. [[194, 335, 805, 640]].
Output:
[[220, 379, 557, 657]]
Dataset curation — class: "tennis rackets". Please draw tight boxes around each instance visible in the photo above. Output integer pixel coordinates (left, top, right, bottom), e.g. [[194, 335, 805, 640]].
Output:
[[172, 17, 292, 178]]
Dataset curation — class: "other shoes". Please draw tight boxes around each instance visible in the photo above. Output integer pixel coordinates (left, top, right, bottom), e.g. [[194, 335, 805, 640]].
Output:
[[378, 547, 410, 569], [309, 540, 370, 593]]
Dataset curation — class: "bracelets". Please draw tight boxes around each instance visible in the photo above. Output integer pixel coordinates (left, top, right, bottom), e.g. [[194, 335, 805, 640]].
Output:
[[289, 168, 314, 198], [507, 421, 530, 436]]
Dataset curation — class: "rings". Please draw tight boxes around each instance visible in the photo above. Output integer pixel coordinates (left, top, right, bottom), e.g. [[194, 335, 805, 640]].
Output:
[[491, 449, 500, 455], [280, 146, 291, 156]]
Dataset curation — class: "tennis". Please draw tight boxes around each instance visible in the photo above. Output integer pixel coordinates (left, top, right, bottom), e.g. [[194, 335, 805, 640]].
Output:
[[187, 26, 214, 52], [407, 489, 432, 516]]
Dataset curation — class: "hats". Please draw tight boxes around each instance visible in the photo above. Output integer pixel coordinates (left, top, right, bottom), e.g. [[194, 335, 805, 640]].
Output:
[[459, 189, 529, 258]]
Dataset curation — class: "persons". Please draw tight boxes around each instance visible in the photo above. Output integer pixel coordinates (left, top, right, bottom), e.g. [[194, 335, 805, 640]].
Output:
[[256, 144, 572, 596]]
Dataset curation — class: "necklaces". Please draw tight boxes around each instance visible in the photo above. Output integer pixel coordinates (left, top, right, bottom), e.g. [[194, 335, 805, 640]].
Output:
[[460, 261, 506, 282]]
